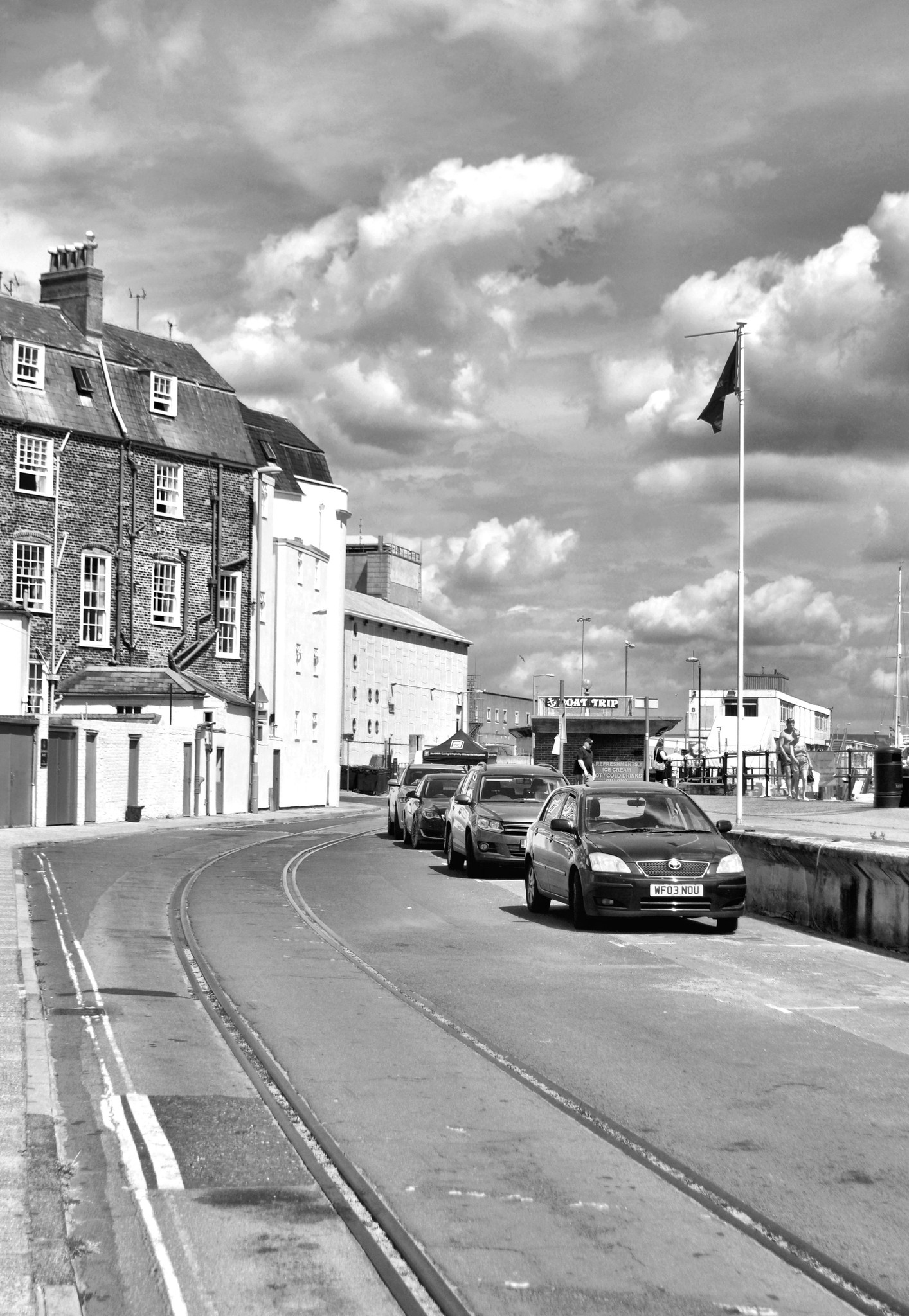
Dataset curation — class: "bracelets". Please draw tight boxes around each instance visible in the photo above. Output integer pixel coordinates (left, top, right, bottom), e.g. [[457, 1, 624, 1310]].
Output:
[[809, 763, 812, 765]]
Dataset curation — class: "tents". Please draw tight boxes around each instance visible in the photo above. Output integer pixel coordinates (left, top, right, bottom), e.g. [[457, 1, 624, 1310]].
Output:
[[422, 729, 488, 771]]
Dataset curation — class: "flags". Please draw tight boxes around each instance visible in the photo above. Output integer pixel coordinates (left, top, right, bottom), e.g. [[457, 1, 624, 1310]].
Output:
[[697, 337, 739, 434]]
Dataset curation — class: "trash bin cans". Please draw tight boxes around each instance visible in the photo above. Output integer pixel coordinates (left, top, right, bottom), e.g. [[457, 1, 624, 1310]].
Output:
[[356, 765, 391, 796], [873, 747, 906, 808], [340, 764, 361, 790]]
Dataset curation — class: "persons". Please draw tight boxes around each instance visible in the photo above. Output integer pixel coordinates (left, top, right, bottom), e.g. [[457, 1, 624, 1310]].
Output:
[[670, 748, 714, 788], [779, 719, 813, 801], [573, 738, 594, 784], [654, 738, 671, 787]]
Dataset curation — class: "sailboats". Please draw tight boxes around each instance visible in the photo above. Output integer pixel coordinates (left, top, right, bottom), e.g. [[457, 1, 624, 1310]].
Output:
[[880, 561, 909, 781]]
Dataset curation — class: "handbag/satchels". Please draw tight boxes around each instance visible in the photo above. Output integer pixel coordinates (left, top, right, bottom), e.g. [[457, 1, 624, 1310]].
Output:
[[807, 768, 815, 783], [653, 747, 665, 770]]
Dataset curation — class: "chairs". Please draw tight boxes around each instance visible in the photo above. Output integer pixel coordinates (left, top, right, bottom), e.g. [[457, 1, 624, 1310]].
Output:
[[430, 781, 447, 796], [529, 778, 549, 799], [409, 773, 423, 786], [485, 781, 501, 799], [500, 788, 515, 800], [523, 788, 533, 798], [643, 809, 670, 828], [586, 800, 603, 828]]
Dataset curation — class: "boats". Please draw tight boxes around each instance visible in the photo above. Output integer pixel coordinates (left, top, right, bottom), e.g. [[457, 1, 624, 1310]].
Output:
[[644, 664, 878, 797]]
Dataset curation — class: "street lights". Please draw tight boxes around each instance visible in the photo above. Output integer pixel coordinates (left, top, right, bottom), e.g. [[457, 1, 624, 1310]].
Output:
[[249, 462, 284, 813], [576, 615, 592, 696], [685, 657, 702, 782], [873, 730, 880, 749], [624, 640, 635, 717], [532, 674, 555, 715], [837, 722, 851, 739]]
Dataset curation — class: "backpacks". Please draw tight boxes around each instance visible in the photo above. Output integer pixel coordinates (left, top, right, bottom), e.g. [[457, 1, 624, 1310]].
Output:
[[683, 756, 689, 765]]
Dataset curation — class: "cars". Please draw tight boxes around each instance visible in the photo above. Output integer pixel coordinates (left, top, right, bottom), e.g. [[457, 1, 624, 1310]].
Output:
[[402, 773, 467, 847], [524, 780, 746, 933]]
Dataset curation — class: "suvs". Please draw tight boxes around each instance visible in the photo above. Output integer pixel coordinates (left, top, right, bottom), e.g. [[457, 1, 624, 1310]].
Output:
[[387, 762, 468, 840], [442, 762, 571, 877]]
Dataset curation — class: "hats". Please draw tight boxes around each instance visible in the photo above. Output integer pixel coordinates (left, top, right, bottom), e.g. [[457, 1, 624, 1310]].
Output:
[[787, 719, 794, 722], [585, 738, 594, 744]]
